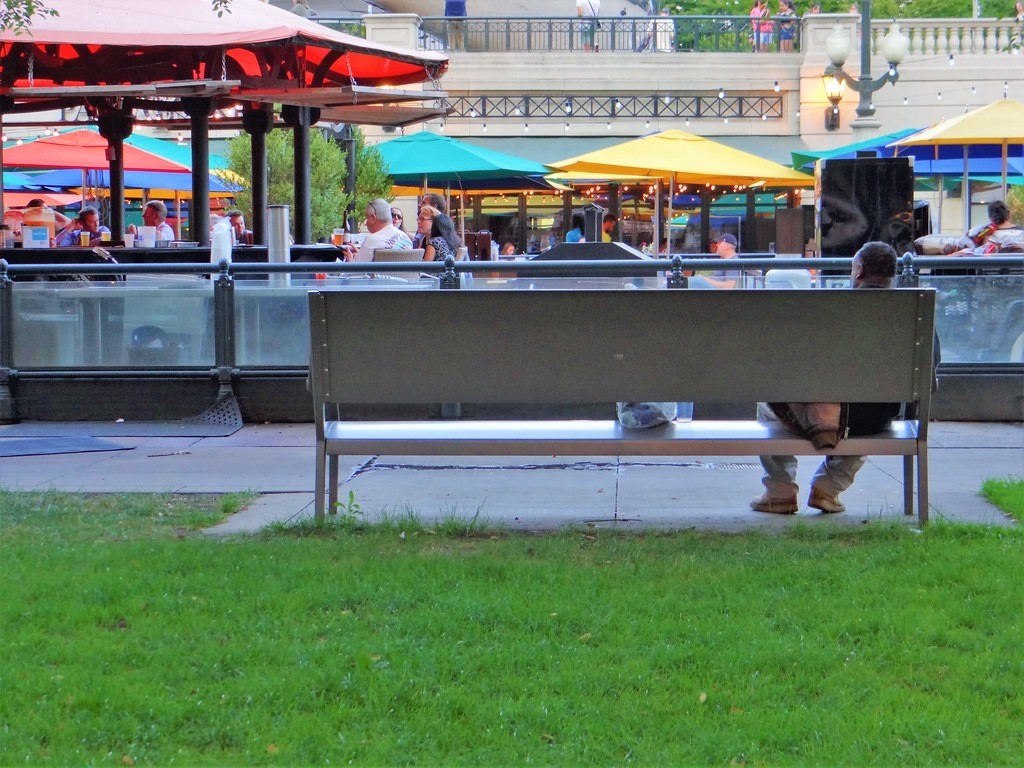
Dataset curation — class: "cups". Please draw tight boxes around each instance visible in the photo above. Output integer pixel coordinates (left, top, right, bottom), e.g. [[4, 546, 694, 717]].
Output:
[[81, 232, 90, 246], [316, 273, 327, 286], [137, 226, 156, 248], [334, 228, 345, 245], [4, 230, 14, 249], [124, 234, 135, 247], [102, 232, 111, 241], [676, 402, 694, 422], [247, 233, 253, 245]]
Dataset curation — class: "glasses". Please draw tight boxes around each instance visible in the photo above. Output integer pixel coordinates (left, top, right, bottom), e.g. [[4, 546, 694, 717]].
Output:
[[417, 216, 433, 223], [391, 213, 403, 221], [367, 201, 376, 216]]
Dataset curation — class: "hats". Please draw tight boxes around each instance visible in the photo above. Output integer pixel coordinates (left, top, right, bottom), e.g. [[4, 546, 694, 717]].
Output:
[[713, 233, 738, 247]]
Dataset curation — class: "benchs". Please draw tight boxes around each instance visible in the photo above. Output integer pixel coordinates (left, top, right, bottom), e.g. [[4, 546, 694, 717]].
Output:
[[305, 283, 938, 529]]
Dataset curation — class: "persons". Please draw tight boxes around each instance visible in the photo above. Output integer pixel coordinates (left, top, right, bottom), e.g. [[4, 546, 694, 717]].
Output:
[[749, 243, 942, 513], [27, 192, 741, 291], [576, 0, 600, 53], [444, 0, 467, 52], [648, 7, 674, 52], [290, 0, 316, 23], [987, 200, 1023, 243], [1010, 1, 1024, 54], [750, 0, 862, 53]]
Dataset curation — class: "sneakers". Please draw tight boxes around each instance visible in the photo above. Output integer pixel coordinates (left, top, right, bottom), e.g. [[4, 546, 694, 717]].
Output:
[[750, 490, 798, 514], [807, 487, 846, 511]]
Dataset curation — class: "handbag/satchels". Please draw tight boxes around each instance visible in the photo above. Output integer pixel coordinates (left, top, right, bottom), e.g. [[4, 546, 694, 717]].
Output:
[[616, 400, 678, 430]]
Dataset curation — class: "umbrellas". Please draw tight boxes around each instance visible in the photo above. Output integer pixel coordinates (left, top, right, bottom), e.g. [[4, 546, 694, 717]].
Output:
[[1, 131, 1024, 242]]
